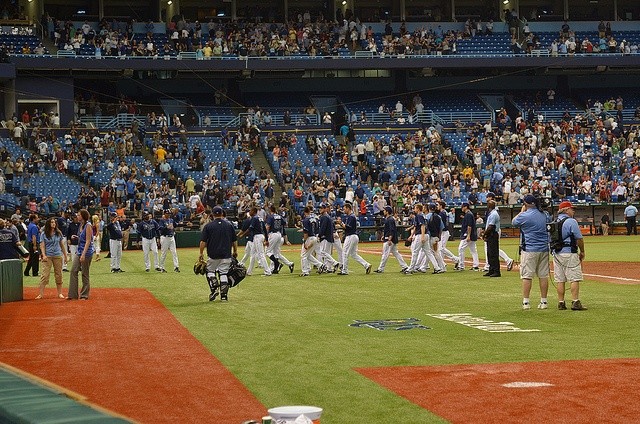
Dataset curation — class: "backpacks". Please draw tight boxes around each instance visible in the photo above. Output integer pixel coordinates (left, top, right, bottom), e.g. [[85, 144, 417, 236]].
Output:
[[544, 217, 570, 254]]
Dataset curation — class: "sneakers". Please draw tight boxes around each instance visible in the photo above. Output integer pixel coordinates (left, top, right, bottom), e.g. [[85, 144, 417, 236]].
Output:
[[432, 269, 441, 274], [337, 272, 348, 275], [275, 263, 283, 272], [208, 291, 219, 301], [457, 267, 465, 271], [373, 270, 384, 273], [58, 294, 65, 299], [116, 268, 125, 272], [416, 268, 426, 273], [35, 294, 43, 300], [521, 302, 531, 310], [557, 301, 567, 310], [174, 267, 180, 273], [33, 273, 41, 276], [469, 267, 479, 271], [111, 268, 118, 273], [62, 269, 70, 272], [299, 273, 308, 276], [146, 269, 150, 272], [365, 265, 372, 274], [453, 260, 459, 270], [571, 300, 588, 310], [333, 263, 339, 273], [324, 270, 332, 274], [537, 302, 548, 309], [161, 269, 167, 273], [289, 262, 294, 273], [104, 253, 111, 258], [400, 267, 408, 272], [79, 297, 88, 300], [155, 268, 161, 271], [65, 297, 78, 300], [221, 296, 227, 301], [507, 259, 514, 271], [318, 264, 326, 275]]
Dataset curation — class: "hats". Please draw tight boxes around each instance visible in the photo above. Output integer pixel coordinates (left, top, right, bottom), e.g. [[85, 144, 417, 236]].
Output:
[[282, 192, 288, 196], [524, 195, 536, 204], [428, 203, 436, 211], [212, 206, 223, 216], [462, 203, 469, 207], [268, 207, 276, 213], [485, 192, 495, 198], [383, 206, 392, 215], [559, 201, 577, 210]]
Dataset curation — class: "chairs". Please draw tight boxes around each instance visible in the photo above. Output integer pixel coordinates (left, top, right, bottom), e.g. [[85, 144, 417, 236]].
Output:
[[433, 31, 565, 56], [131, 31, 177, 59], [584, 94, 640, 120], [349, 130, 496, 209], [336, 95, 413, 126], [142, 135, 270, 218], [244, 98, 319, 127], [54, 29, 120, 59], [356, 32, 410, 57], [574, 30, 640, 55], [32, 134, 186, 223], [440, 128, 640, 202], [412, 92, 494, 124], [191, 103, 238, 130], [0, 32, 52, 62], [0, 134, 121, 226], [504, 91, 586, 125], [255, 133, 395, 226]]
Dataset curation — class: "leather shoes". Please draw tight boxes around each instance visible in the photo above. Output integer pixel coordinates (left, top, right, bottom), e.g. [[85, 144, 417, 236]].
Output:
[[490, 272, 500, 277], [483, 273, 492, 276]]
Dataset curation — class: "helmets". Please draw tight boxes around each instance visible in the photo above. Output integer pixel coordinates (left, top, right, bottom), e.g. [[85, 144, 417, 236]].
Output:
[[142, 211, 149, 216], [317, 204, 326, 209], [194, 260, 207, 276], [163, 209, 172, 214], [110, 212, 117, 217]]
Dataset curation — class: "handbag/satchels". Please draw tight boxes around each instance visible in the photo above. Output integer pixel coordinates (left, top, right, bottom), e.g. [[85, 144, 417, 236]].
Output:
[[227, 256, 247, 288]]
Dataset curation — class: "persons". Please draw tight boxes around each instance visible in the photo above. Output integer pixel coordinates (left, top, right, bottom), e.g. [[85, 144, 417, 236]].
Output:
[[91, 215, 102, 261], [393, 94, 424, 125], [199, 205, 238, 302], [500, 8, 540, 58], [432, 5, 443, 22], [19, 193, 74, 216], [379, 103, 387, 113], [0, 6, 45, 63], [145, 111, 188, 159], [480, 192, 514, 272], [6, 108, 60, 147], [66, 209, 96, 301], [323, 111, 332, 128], [482, 200, 501, 277], [150, 141, 173, 222], [455, 203, 480, 272], [454, 106, 640, 205], [306, 105, 315, 114], [548, 201, 588, 310], [546, 89, 555, 106], [547, 20, 638, 57], [97, 156, 155, 221], [41, 10, 160, 57], [347, 110, 357, 124], [295, 116, 311, 126], [99, 216, 105, 252], [340, 121, 480, 206], [162, 40, 171, 55], [11, 208, 23, 219], [305, 134, 350, 166], [298, 202, 343, 276], [169, 18, 202, 52], [391, 204, 414, 225], [512, 195, 552, 309], [34, 218, 69, 299], [216, 155, 275, 229], [214, 84, 228, 106], [265, 130, 303, 170], [221, 105, 272, 157], [600, 212, 610, 236], [0, 218, 22, 260], [366, 21, 462, 58], [186, 144, 206, 171], [283, 111, 291, 127], [586, 95, 624, 111], [56, 210, 69, 272], [106, 212, 125, 273], [74, 186, 97, 216], [475, 212, 483, 239], [277, 165, 348, 229], [352, 203, 385, 226], [92, 119, 143, 174], [196, 4, 366, 60], [159, 209, 180, 273], [129, 218, 137, 233], [0, 146, 45, 194], [67, 213, 82, 271], [237, 205, 294, 276], [624, 201, 638, 235], [337, 203, 372, 275], [136, 211, 161, 272], [73, 92, 142, 128], [24, 214, 40, 276], [204, 115, 211, 127], [404, 200, 459, 273], [360, 112, 368, 124], [373, 207, 408, 273], [120, 215, 133, 250], [464, 18, 493, 37], [172, 161, 219, 231], [30, 125, 94, 185]]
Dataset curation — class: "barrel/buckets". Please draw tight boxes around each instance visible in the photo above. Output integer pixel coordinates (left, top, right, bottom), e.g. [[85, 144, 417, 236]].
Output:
[[269, 405, 322, 424]]
